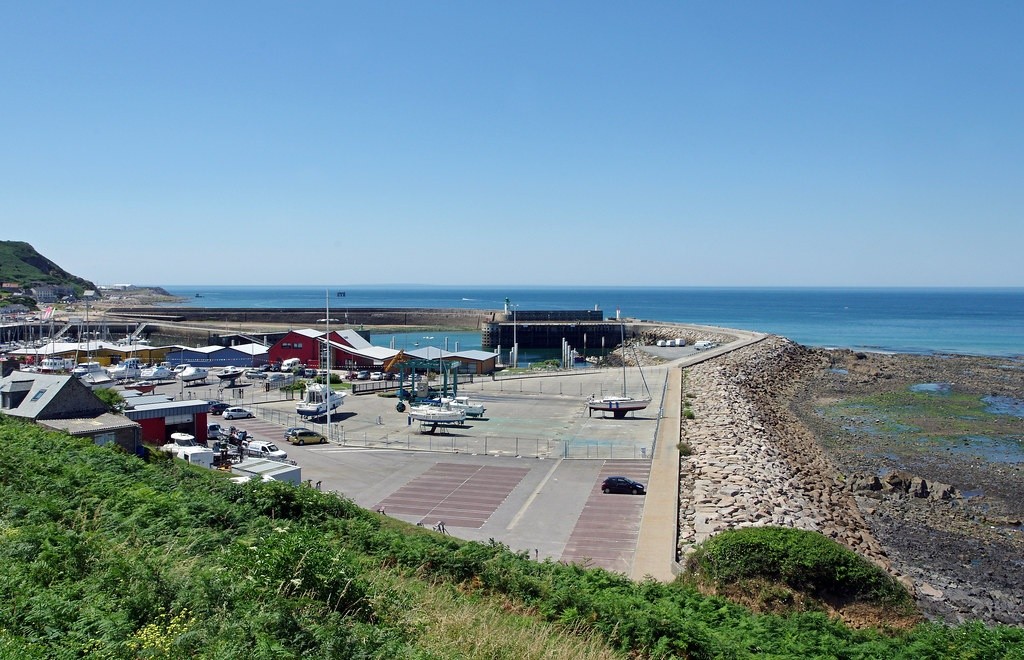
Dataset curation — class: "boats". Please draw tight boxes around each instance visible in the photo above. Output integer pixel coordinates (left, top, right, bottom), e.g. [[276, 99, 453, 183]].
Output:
[[215, 365, 244, 381], [570, 348, 585, 362], [141, 365, 179, 379], [423, 337, 434, 339], [107, 356, 147, 378], [177, 367, 208, 381], [124, 381, 156, 393], [295, 383, 348, 416], [0, 296, 119, 391], [428, 395, 484, 417]]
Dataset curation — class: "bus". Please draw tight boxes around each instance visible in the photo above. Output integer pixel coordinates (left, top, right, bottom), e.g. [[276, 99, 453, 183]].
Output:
[[280, 358, 301, 372]]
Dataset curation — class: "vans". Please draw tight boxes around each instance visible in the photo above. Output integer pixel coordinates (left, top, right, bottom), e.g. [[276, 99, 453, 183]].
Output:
[[656, 338, 686, 347], [247, 441, 288, 461], [357, 370, 370, 380], [694, 340, 716, 350], [207, 421, 221, 440], [304, 369, 317, 376]]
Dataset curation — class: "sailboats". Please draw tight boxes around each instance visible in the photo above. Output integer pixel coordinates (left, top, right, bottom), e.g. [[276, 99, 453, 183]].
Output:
[[407, 344, 466, 423], [587, 316, 653, 411]]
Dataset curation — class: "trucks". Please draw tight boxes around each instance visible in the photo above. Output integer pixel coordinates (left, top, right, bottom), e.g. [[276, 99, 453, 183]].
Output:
[[174, 364, 191, 372]]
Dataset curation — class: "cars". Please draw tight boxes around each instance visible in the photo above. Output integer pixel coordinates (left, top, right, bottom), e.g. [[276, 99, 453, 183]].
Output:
[[205, 400, 220, 412], [245, 369, 268, 379], [601, 476, 645, 496], [210, 403, 232, 415], [259, 364, 270, 372], [221, 407, 254, 420], [370, 372, 383, 381], [317, 371, 332, 377], [284, 427, 328, 445], [270, 364, 280, 372], [382, 373, 391, 379]]
